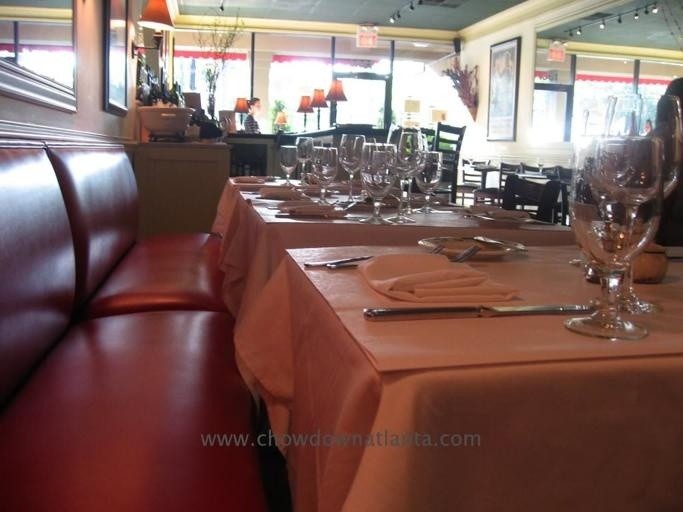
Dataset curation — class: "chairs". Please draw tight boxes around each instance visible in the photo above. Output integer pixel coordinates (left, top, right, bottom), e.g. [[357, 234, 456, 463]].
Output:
[[432, 121, 467, 204], [499, 160, 575, 204], [0, 145, 274, 512], [455, 158, 491, 205], [502, 175, 561, 224], [45, 142, 233, 309]]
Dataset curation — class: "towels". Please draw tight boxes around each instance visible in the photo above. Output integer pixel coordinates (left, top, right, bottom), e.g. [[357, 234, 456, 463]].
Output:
[[496, 209, 530, 218], [278, 199, 333, 214], [259, 186, 301, 199], [359, 251, 521, 302], [234, 176, 268, 190]]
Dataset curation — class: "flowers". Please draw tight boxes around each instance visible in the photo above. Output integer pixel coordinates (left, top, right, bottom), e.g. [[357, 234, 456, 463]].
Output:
[[442, 58, 480, 120]]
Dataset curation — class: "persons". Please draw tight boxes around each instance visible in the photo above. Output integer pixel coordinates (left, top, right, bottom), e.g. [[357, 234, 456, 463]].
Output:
[[244, 98, 261, 134], [645, 120, 653, 135], [653, 77, 683, 247]]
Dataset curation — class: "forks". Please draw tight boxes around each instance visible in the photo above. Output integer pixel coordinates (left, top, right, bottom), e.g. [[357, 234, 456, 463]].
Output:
[[303, 245, 445, 267], [326, 245, 482, 269]]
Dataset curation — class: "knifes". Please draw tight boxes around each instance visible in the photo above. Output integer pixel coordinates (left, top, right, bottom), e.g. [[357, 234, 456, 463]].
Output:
[[276, 214, 347, 219], [363, 304, 595, 322]]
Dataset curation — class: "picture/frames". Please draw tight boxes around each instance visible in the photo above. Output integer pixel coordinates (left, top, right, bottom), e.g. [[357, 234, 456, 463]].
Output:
[[485, 37, 521, 142], [102, 0, 132, 118]]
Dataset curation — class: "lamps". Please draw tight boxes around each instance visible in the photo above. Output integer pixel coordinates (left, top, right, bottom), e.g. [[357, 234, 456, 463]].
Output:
[[295, 95, 314, 131], [404, 98, 421, 128], [388, 14, 397, 24], [652, 2, 658, 14], [311, 88, 329, 130], [633, 9, 641, 20], [600, 18, 605, 30], [430, 109, 446, 123], [136, 0, 177, 36], [325, 81, 348, 126], [234, 97, 250, 133], [576, 26, 582, 33]]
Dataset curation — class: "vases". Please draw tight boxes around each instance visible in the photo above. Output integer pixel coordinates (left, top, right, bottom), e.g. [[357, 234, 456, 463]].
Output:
[[468, 108, 478, 123]]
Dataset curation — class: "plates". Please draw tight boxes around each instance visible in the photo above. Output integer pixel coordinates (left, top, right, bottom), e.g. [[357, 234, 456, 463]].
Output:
[[418, 236, 513, 261], [460, 209, 487, 217], [475, 211, 535, 228]]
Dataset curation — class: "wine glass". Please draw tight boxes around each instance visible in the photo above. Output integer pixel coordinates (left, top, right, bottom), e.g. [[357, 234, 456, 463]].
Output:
[[361, 143, 397, 225], [568, 94, 683, 340], [339, 135, 378, 204], [386, 124, 443, 223], [297, 137, 314, 185], [280, 145, 299, 186], [312, 147, 338, 207]]
